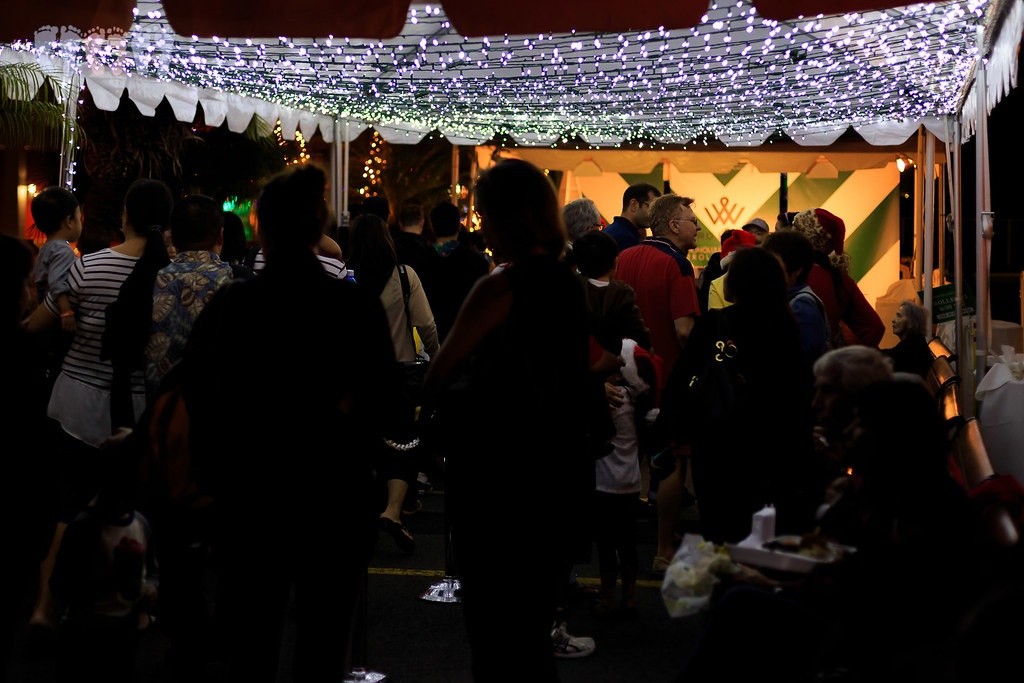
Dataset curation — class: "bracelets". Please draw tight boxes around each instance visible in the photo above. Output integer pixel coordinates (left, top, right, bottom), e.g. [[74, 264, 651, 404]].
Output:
[[60, 311, 74, 318]]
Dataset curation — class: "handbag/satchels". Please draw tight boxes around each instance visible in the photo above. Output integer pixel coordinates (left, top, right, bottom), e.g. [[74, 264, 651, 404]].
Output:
[[692, 309, 737, 424], [399, 359, 431, 389]]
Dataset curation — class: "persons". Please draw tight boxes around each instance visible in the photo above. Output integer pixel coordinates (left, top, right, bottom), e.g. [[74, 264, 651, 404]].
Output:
[[0, 161, 1024, 683]]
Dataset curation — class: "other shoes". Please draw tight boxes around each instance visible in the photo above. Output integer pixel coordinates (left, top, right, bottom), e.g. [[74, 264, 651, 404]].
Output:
[[82, 602, 160, 633], [378, 512, 416, 554], [549, 622, 596, 658]]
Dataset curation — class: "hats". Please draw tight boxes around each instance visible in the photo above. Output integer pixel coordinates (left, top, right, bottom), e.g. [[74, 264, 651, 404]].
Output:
[[720, 230, 758, 270], [742, 219, 769, 232], [795, 206, 850, 269]]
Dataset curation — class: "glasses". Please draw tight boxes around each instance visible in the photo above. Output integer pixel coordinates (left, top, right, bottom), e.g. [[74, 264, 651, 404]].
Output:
[[675, 217, 697, 225], [590, 222, 604, 230]]
